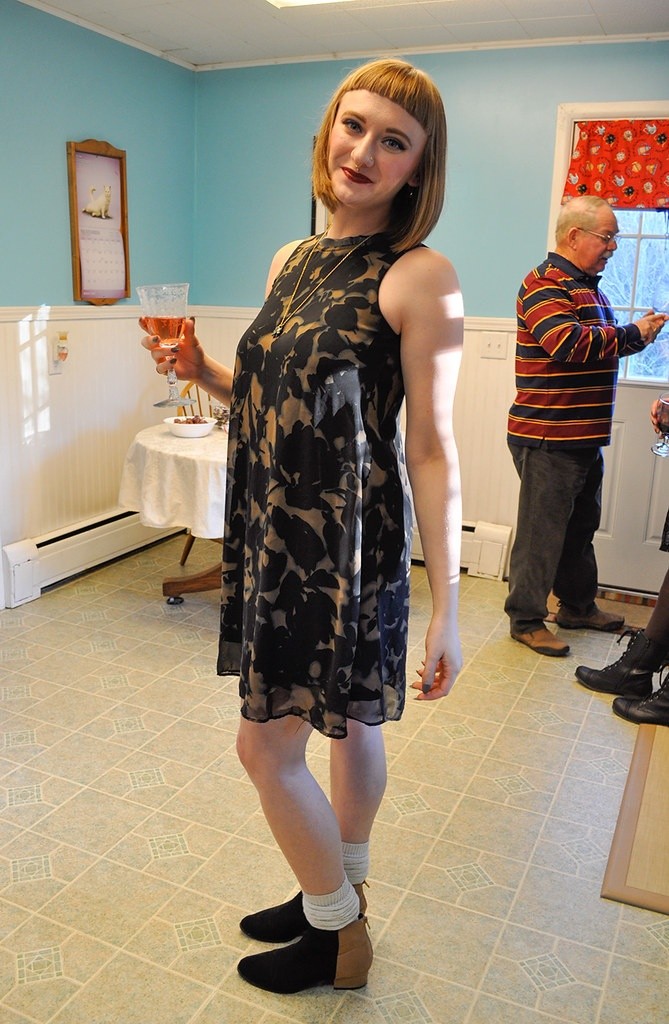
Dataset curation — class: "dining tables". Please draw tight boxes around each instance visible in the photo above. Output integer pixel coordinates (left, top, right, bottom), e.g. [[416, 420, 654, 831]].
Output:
[[117, 416, 229, 605]]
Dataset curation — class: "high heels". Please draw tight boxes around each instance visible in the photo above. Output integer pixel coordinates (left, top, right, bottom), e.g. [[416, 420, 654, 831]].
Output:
[[237, 913, 372, 995], [240, 880, 370, 943]]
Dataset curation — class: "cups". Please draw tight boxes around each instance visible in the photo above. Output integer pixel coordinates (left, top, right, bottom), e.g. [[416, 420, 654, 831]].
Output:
[[652, 277, 669, 315]]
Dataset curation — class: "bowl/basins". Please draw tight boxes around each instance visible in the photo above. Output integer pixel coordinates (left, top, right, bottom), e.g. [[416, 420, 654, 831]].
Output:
[[164, 417, 218, 438]]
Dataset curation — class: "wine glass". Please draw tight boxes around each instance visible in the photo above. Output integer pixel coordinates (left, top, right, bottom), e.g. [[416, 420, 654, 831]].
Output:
[[135, 283, 197, 408], [649, 392, 669, 458]]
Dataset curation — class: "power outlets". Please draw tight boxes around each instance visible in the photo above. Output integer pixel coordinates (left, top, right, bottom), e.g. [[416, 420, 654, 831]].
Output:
[[47, 337, 63, 375]]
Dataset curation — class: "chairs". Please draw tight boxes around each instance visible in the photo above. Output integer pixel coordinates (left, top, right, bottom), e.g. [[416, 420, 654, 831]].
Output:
[[177, 382, 223, 565]]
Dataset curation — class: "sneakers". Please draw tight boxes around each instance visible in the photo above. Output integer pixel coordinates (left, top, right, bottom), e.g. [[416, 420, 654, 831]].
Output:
[[556, 605, 625, 632], [510, 627, 570, 656]]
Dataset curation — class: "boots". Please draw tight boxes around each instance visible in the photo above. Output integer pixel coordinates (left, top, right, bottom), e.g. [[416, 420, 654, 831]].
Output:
[[612, 673, 669, 727], [574, 629, 669, 699]]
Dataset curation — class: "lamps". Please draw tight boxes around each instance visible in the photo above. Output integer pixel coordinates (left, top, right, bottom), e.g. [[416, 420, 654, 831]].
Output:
[[54, 331, 71, 363]]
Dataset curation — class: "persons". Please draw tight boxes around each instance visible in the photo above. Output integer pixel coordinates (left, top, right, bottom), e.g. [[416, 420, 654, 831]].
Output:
[[137, 58, 465, 993], [504, 196, 669, 657], [575, 392, 669, 727]]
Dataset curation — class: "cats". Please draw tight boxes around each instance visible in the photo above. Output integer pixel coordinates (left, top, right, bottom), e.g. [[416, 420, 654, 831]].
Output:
[[81, 185, 112, 219]]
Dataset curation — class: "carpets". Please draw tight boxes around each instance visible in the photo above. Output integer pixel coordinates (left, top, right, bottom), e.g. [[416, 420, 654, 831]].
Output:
[[601, 724, 669, 916], [545, 590, 654, 634]]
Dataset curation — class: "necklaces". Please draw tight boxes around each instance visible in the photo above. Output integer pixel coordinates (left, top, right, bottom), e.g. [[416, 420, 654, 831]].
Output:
[[272, 223, 379, 337]]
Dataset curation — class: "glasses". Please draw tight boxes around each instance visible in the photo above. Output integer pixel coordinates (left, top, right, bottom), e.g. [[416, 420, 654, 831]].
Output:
[[576, 227, 622, 245]]
